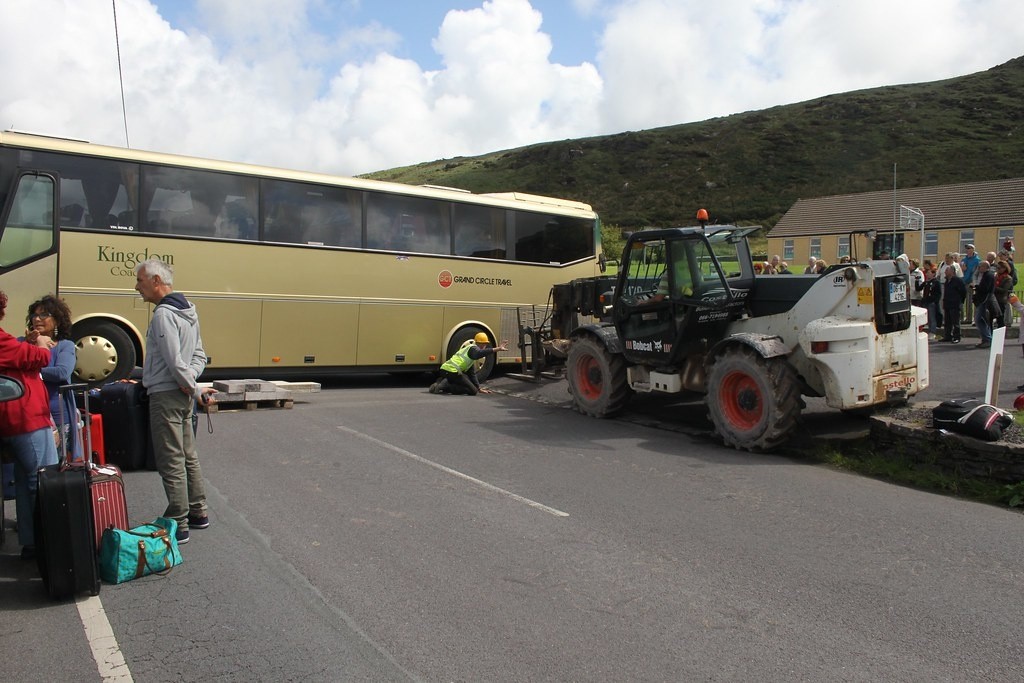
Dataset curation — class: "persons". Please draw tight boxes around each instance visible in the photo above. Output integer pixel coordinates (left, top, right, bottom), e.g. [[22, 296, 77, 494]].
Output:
[[0, 291, 60, 562], [133, 259, 217, 544], [636, 244, 709, 303], [428, 332, 512, 395], [753, 236, 1024, 357], [2, 296, 85, 529]]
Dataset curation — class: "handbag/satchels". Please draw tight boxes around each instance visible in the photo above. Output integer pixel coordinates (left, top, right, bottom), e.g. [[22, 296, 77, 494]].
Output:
[[932, 398, 1014, 442], [101, 517, 184, 584]]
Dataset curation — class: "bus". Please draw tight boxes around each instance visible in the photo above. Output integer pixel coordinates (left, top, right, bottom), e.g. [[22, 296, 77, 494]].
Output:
[[0, 129, 611, 386]]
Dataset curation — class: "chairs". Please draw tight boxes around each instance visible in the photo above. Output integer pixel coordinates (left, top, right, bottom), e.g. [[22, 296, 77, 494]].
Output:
[[42, 203, 255, 240]]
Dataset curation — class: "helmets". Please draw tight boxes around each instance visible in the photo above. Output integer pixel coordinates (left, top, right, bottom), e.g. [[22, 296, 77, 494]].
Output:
[[475, 333, 489, 343]]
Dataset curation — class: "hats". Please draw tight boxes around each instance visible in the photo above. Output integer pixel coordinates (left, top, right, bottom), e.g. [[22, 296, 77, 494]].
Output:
[[754, 264, 762, 272]]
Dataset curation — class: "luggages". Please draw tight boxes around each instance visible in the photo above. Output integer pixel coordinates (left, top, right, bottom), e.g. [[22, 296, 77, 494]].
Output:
[[38, 382, 129, 597], [74, 380, 199, 472]]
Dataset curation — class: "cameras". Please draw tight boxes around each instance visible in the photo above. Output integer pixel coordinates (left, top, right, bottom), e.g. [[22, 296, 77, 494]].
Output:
[[201, 392, 215, 403], [918, 276, 921, 278]]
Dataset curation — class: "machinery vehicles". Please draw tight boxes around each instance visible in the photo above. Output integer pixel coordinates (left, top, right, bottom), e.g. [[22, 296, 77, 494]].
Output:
[[506, 209, 930, 451]]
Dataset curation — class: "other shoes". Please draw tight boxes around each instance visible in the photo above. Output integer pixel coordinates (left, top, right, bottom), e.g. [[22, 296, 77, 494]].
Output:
[[928, 333, 936, 339], [175, 531, 190, 544], [976, 341, 990, 348], [1004, 323, 1011, 327], [972, 321, 977, 327], [938, 338, 952, 342], [960, 318, 972, 324], [20, 545, 39, 561], [429, 378, 447, 394], [185, 514, 210, 528], [1017, 384, 1024, 391], [952, 339, 960, 343]]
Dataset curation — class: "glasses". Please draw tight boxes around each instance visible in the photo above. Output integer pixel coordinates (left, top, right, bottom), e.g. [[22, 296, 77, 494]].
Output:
[[31, 312, 52, 320]]
